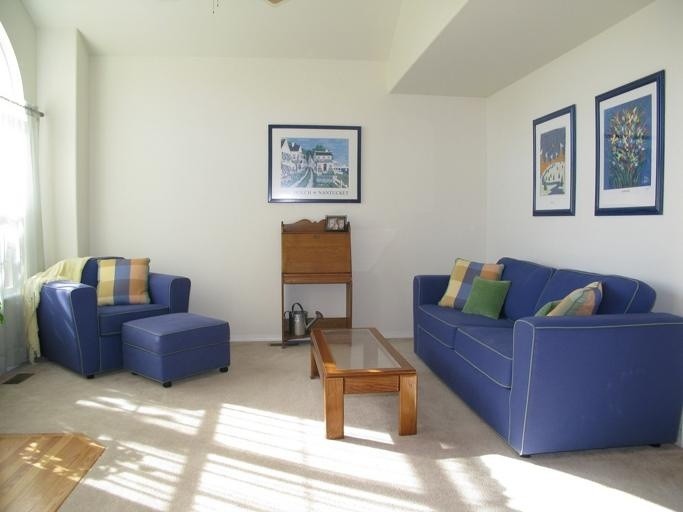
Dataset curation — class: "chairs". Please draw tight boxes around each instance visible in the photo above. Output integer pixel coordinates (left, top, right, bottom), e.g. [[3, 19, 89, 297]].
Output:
[[33, 257, 191, 379]]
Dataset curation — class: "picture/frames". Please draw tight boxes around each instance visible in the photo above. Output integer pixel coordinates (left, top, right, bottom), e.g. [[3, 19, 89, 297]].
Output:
[[268, 124, 365, 203], [595, 70, 663, 216], [533, 103, 575, 215], [324, 214, 348, 232]]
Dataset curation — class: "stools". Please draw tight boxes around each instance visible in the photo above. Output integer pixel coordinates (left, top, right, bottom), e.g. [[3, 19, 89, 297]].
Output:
[[121, 312, 230, 388]]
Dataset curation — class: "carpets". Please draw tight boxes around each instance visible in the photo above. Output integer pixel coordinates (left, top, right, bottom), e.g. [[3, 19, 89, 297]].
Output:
[[0, 433, 107, 512]]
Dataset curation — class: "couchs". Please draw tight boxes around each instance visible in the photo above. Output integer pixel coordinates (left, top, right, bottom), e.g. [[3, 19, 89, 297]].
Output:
[[412, 257, 683, 458]]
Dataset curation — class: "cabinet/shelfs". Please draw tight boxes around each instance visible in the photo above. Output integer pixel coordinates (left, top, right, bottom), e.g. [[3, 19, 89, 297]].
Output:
[[280, 217, 353, 349]]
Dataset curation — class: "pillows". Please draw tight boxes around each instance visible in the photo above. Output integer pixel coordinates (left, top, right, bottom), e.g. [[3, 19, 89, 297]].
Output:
[[546, 280, 604, 317], [535, 301, 558, 317], [97, 258, 152, 306], [438, 258, 504, 309], [461, 276, 512, 320]]
